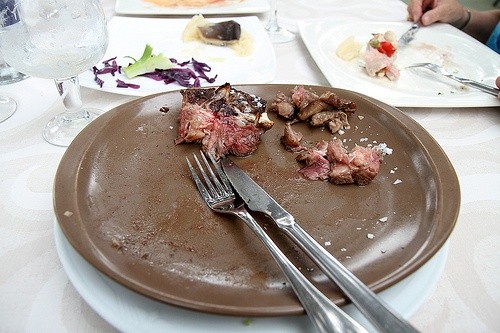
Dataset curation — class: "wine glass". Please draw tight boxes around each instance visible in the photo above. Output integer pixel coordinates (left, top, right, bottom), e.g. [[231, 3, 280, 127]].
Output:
[[0, 0, 109, 148]]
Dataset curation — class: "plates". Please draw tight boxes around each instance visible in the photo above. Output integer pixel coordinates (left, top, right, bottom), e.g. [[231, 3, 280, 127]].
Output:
[[296, 18, 500, 109], [115, 0, 269, 15], [77, 15, 279, 98], [50, 82, 462, 331]]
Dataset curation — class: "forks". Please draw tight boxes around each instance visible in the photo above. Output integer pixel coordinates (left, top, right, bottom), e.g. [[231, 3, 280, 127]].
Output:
[[406, 62, 500, 98], [185, 150, 368, 332]]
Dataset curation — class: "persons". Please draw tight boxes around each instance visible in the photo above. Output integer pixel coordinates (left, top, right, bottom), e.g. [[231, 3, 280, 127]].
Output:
[[407, 0, 500, 96]]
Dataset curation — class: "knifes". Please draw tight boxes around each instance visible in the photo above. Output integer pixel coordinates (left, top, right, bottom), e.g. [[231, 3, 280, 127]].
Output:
[[397, 7, 432, 47], [221, 157, 419, 333]]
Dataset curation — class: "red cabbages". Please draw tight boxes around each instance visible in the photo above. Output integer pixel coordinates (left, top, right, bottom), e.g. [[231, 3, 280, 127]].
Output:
[[92, 56, 217, 89]]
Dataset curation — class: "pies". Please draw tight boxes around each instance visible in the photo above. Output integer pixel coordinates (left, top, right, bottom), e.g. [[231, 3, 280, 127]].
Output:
[[143, 0, 244, 8]]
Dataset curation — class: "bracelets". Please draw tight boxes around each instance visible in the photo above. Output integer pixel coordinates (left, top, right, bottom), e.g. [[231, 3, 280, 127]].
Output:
[[458, 8, 471, 30]]
